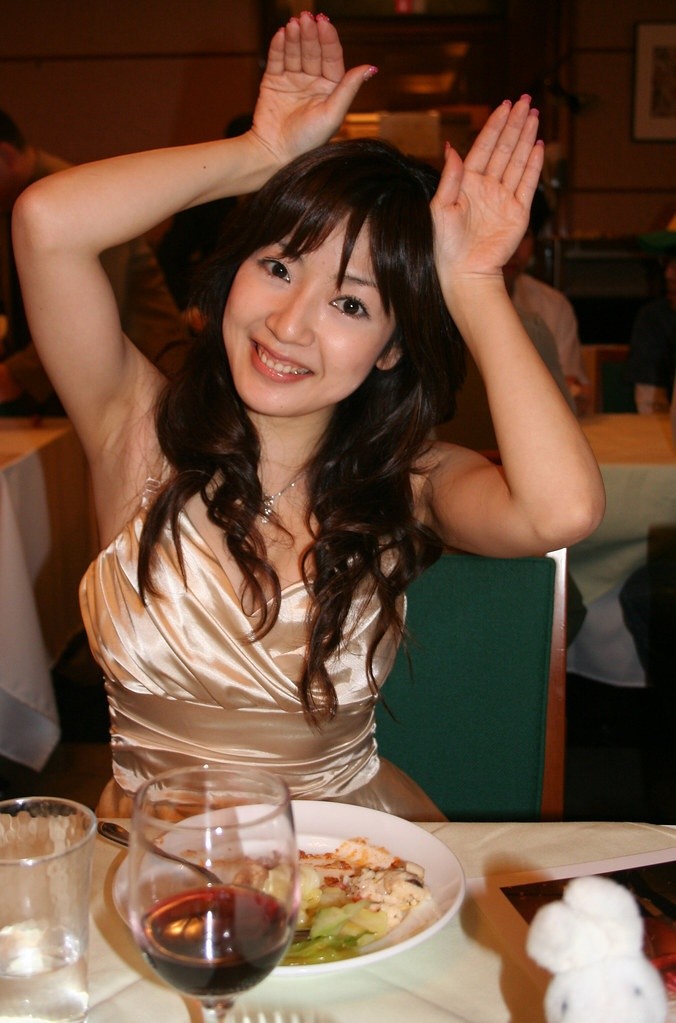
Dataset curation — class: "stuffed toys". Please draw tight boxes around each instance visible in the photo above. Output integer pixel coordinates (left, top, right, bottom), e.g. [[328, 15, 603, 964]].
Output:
[[526, 877, 667, 1023]]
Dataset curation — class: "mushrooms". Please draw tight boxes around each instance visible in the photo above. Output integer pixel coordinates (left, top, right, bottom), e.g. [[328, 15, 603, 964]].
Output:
[[384, 860, 426, 889]]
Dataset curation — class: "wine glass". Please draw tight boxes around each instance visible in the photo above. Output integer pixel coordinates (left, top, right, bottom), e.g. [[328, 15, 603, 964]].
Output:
[[127, 766, 300, 1023]]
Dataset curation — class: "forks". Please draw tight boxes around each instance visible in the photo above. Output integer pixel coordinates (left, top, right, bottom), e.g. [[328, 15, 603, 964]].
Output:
[[97, 820, 310, 945]]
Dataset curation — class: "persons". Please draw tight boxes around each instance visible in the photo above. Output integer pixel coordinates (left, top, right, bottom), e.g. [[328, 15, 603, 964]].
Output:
[[11, 11, 607, 823], [0, 110, 196, 404], [629, 212, 676, 415]]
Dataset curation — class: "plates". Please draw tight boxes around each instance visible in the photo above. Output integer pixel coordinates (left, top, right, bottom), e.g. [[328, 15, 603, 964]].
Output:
[[111, 799, 466, 975]]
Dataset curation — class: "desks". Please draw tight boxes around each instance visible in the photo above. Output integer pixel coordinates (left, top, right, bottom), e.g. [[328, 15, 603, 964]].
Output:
[[0, 812, 676, 1023], [552, 409, 676, 694], [0, 418, 99, 776]]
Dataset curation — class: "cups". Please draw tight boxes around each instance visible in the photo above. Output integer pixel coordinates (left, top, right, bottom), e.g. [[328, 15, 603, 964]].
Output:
[[0, 797, 97, 1023]]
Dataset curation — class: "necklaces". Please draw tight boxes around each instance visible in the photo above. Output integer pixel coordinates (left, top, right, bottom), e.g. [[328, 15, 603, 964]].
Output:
[[256, 473, 302, 524]]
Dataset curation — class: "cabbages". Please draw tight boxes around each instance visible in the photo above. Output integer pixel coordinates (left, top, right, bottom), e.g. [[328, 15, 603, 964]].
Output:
[[262, 863, 388, 966]]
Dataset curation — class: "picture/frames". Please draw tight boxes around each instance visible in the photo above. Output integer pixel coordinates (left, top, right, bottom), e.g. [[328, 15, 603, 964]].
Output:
[[631, 20, 675, 142]]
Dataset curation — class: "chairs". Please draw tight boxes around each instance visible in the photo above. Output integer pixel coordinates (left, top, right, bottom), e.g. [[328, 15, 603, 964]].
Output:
[[372, 542, 566, 823]]
